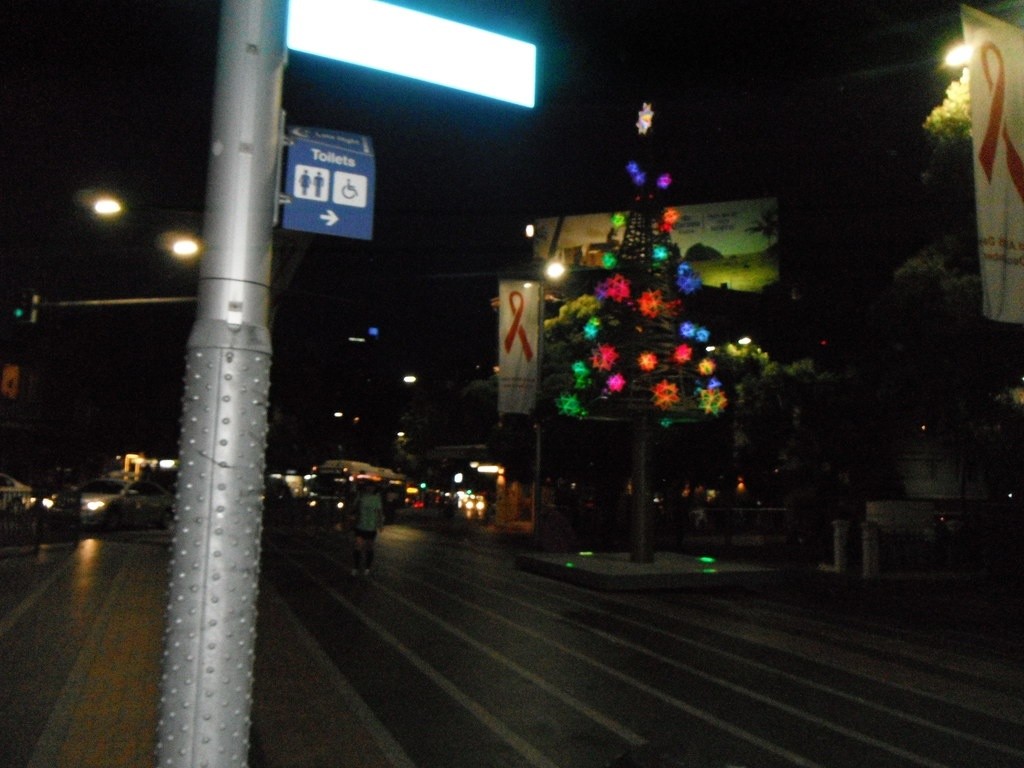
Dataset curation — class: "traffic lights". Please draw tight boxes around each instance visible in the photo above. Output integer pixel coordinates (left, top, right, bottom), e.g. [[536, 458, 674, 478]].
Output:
[[11, 299, 29, 324]]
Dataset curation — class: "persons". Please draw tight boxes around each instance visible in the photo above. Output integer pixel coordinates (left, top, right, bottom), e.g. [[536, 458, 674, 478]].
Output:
[[350, 483, 384, 577], [52, 477, 81, 551]]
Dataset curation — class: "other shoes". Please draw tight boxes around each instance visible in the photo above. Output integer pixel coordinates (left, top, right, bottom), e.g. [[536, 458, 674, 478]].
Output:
[[351, 567, 357, 577], [364, 567, 369, 576]]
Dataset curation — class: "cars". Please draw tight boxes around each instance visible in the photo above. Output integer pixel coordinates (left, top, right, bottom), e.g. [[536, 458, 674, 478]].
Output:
[[264, 469, 493, 526], [39, 477, 178, 533]]
